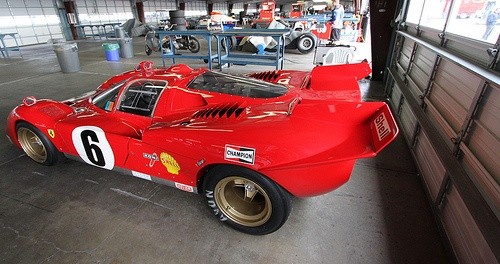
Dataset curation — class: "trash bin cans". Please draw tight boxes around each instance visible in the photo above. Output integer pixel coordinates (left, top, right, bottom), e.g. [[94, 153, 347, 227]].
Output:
[[117, 38, 133, 58], [102, 43, 120, 61], [51, 44, 80, 73]]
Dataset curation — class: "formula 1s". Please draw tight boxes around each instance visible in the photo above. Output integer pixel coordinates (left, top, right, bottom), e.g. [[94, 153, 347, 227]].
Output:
[[6, 60, 399, 236]]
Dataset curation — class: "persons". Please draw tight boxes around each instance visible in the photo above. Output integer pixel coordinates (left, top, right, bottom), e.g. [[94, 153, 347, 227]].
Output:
[[481, 8, 500, 40], [326, 0, 344, 46]]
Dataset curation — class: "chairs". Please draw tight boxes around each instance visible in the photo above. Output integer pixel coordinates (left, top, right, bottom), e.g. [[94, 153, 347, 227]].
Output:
[[323, 47, 355, 66]]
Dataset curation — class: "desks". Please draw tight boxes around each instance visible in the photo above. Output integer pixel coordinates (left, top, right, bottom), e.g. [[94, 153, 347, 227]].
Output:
[[75, 23, 121, 40], [154, 30, 221, 70], [284, 12, 358, 44], [212, 29, 290, 71], [0, 33, 22, 56]]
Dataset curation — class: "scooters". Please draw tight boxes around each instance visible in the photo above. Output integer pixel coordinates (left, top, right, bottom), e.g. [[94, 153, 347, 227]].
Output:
[[145, 24, 200, 56]]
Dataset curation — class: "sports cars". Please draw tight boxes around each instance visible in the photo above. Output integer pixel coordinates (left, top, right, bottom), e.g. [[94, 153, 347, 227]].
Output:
[[221, 19, 317, 54]]
